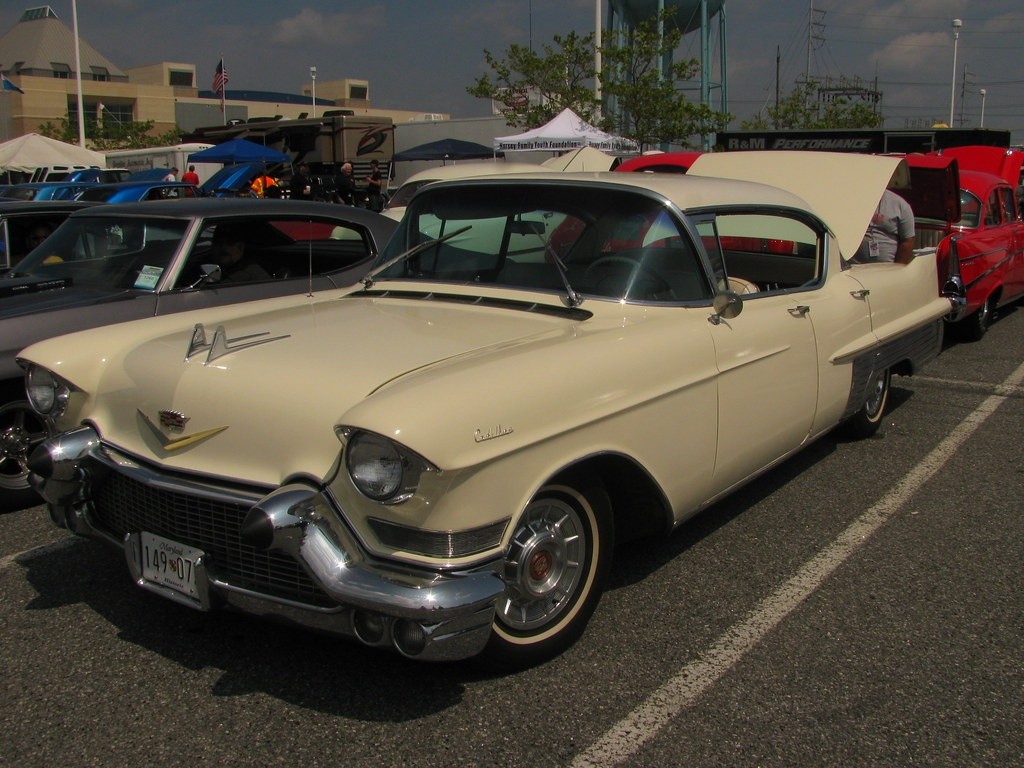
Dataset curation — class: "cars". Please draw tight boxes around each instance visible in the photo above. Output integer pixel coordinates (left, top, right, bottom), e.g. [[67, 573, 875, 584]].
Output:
[[0, 146, 1024, 341], [15, 147, 954, 661]]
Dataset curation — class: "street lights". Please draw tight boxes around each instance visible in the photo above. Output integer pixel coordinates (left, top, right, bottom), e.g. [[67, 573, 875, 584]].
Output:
[[980, 88, 988, 127], [310, 67, 316, 117], [951, 18, 962, 130]]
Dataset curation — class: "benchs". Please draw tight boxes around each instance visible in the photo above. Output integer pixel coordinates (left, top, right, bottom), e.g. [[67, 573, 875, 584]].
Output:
[[616, 242, 816, 300]]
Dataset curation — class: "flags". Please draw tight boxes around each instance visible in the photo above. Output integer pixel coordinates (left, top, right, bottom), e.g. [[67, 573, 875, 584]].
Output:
[[97, 99, 104, 129], [0, 74, 25, 94], [212, 58, 228, 111]]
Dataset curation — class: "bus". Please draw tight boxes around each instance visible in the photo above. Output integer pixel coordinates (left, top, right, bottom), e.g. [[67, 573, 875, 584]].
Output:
[[181, 109, 396, 189], [105, 143, 224, 186]]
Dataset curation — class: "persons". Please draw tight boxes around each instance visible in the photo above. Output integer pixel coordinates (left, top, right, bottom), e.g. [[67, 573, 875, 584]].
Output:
[[181, 166, 198, 195], [0, 224, 67, 291], [164, 168, 179, 198], [250, 168, 282, 200], [366, 160, 382, 212], [849, 190, 916, 265], [291, 162, 355, 207], [169, 223, 274, 285]]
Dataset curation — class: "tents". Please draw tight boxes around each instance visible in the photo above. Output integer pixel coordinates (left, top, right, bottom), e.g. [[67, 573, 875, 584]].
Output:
[[0, 133, 106, 184], [494, 107, 639, 163], [184, 139, 296, 178], [387, 139, 506, 186]]
